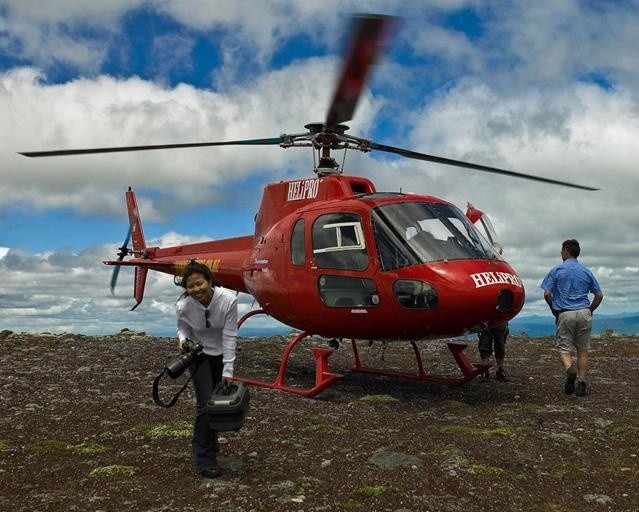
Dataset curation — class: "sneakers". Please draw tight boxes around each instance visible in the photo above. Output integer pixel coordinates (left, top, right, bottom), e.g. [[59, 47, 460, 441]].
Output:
[[565, 367, 588, 396], [479, 367, 508, 382], [215, 436, 219, 452], [201, 463, 221, 478]]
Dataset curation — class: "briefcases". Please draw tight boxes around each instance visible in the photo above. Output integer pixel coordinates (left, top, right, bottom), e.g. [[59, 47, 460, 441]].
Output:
[[207, 381, 249, 432]]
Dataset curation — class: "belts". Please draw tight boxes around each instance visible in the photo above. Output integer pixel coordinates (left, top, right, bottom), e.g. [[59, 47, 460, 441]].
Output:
[[560, 307, 589, 313]]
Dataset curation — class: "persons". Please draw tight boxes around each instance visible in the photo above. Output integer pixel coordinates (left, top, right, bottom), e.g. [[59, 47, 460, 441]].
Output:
[[478, 321, 509, 381], [540, 240, 603, 396], [178, 259, 238, 478]]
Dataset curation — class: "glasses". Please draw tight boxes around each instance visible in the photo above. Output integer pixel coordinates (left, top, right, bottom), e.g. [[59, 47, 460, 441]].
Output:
[[205, 310, 211, 328]]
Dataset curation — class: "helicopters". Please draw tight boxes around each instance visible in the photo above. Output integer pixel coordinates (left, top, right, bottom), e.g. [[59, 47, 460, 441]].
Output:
[[15, 13, 603, 395]]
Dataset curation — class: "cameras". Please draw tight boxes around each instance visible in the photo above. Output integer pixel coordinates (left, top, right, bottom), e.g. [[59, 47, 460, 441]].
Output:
[[166, 338, 205, 379]]
